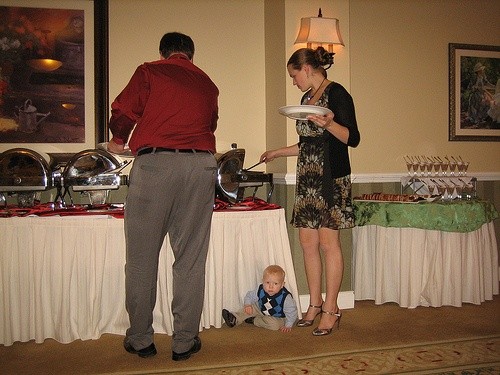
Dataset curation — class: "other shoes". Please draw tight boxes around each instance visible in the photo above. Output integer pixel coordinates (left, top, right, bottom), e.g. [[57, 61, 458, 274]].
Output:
[[222, 309, 236, 328], [245, 317, 254, 324]]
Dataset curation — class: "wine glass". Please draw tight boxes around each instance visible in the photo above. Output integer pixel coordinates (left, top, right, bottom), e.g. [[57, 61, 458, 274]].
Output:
[[428, 185, 474, 204], [406, 162, 470, 177]]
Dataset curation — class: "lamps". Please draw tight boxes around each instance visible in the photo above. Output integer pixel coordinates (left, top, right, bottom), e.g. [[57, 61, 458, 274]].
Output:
[[294, 8, 345, 53]]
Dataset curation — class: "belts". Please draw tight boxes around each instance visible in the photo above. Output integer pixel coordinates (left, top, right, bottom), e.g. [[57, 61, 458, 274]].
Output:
[[137, 146, 213, 156]]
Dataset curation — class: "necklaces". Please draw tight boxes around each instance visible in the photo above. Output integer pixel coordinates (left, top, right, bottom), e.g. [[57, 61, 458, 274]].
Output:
[[308, 77, 326, 101]]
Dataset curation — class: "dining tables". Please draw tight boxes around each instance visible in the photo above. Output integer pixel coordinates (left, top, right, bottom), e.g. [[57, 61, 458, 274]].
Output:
[[351, 201, 500, 308], [0, 203, 302, 346]]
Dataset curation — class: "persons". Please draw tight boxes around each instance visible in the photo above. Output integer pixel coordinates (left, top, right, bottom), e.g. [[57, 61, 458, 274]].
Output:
[[258, 46, 362, 336], [222, 265, 297, 333], [107, 31, 220, 361]]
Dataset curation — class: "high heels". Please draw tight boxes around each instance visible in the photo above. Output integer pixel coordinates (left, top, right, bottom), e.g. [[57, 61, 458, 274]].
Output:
[[296, 301, 326, 327], [312, 308, 343, 336]]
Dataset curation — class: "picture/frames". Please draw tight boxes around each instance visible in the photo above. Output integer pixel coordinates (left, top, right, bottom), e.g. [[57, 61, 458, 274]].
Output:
[[0, 0, 109, 161], [448, 42, 500, 142]]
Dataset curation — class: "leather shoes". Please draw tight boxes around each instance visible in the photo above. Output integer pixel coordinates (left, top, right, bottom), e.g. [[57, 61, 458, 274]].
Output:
[[171, 336, 202, 361], [124, 337, 157, 359]]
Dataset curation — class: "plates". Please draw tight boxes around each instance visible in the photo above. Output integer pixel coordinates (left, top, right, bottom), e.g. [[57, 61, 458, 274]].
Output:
[[419, 198, 435, 203], [99, 142, 135, 158], [278, 105, 332, 121], [435, 200, 458, 205], [354, 199, 421, 203], [46, 153, 77, 161]]
